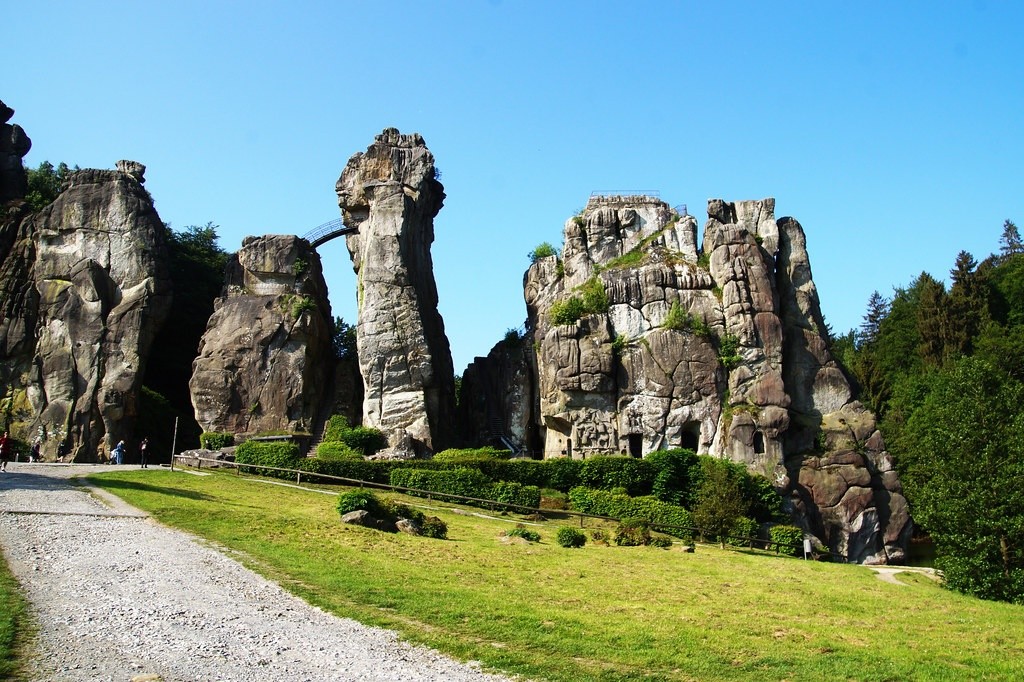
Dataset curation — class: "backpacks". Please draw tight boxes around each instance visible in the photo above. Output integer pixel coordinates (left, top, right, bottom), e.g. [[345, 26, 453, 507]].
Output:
[[141, 442, 148, 451]]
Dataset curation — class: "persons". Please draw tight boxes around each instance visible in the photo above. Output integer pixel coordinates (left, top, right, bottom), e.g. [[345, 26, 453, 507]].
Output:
[[34, 441, 41, 463], [139, 436, 150, 468], [116, 440, 126, 465], [57, 439, 66, 463], [0, 430, 10, 472]]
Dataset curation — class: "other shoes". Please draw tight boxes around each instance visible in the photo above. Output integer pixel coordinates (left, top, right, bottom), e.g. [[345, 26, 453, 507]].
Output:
[[145, 466, 147, 468], [141, 466, 143, 468]]
